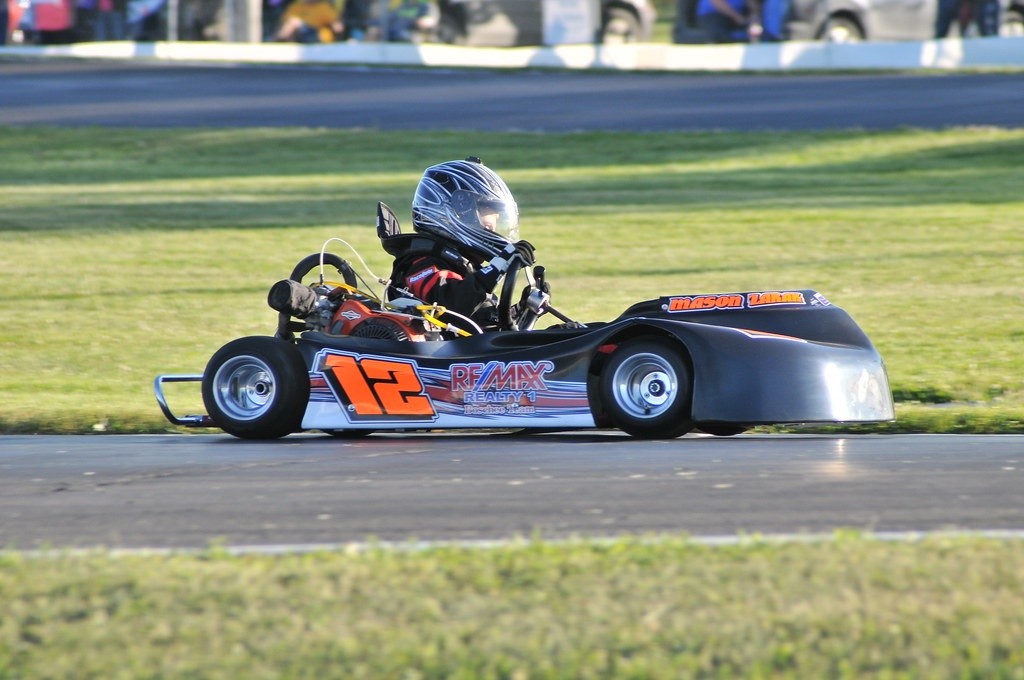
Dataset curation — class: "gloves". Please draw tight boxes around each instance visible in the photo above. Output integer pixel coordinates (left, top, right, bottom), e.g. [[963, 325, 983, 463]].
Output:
[[510, 281, 552, 328], [488, 240, 536, 275]]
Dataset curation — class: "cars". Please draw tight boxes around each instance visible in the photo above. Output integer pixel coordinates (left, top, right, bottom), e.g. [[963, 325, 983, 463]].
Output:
[[420, 0, 655, 50], [783, 0, 939, 44]]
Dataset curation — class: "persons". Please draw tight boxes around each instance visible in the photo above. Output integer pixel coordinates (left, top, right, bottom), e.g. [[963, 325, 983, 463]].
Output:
[[277, 0, 343, 43], [376, 156, 552, 341], [367, 0, 441, 44]]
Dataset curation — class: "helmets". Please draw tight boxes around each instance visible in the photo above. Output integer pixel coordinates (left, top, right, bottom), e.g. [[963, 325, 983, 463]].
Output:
[[411, 156, 518, 263]]
